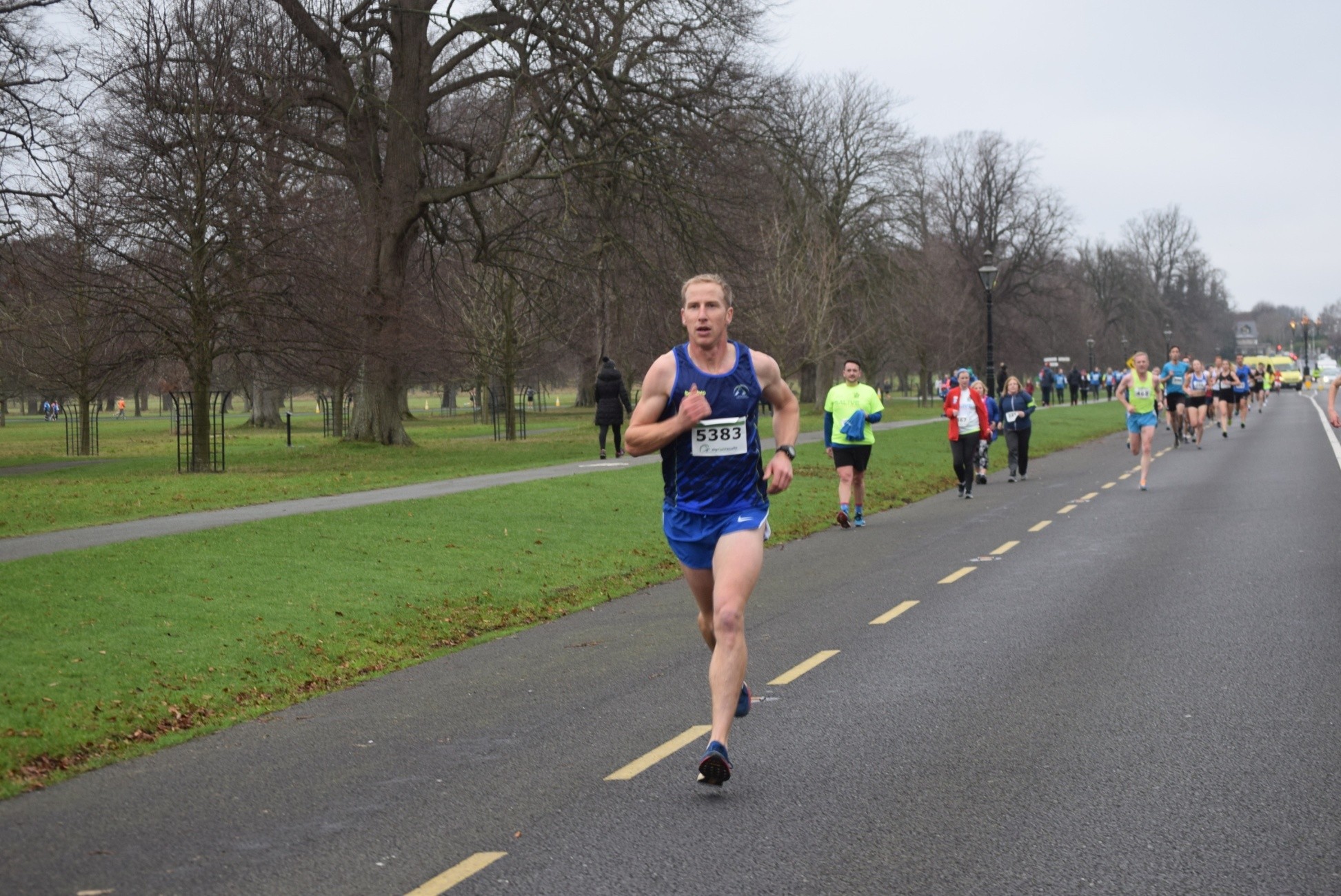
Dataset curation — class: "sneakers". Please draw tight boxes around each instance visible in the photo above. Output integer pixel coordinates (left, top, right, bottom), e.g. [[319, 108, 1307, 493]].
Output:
[[696, 741, 734, 786], [734, 681, 750, 718], [837, 510, 850, 528], [853, 513, 865, 526]]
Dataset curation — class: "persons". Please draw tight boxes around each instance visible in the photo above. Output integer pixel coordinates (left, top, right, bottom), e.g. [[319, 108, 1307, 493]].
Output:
[[997, 376, 1035, 482], [625, 274, 800, 785], [1328, 376, 1341, 428], [970, 380, 1000, 485], [996, 346, 1283, 449], [470, 391, 475, 403], [938, 369, 980, 416], [884, 381, 890, 399], [824, 361, 884, 528], [527, 387, 534, 410], [1313, 363, 1319, 383], [1115, 352, 1163, 493], [943, 368, 989, 500], [594, 357, 632, 459]]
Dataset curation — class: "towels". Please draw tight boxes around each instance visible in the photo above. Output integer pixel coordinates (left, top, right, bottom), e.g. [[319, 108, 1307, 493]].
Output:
[[839, 409, 866, 442]]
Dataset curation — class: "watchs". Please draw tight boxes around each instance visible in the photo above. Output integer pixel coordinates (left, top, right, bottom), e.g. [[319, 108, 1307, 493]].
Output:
[[775, 445, 796, 461]]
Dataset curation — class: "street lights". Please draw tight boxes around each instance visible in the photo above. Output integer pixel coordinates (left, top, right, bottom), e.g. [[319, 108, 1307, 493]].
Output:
[[1314, 317, 1323, 336], [1120, 334, 1130, 370], [1289, 317, 1297, 351], [976, 250, 1000, 399], [1086, 333, 1095, 373], [1300, 313, 1311, 377], [1162, 323, 1173, 363]]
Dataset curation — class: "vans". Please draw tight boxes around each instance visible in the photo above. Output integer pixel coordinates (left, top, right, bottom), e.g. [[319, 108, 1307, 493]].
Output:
[[1242, 356, 1304, 392]]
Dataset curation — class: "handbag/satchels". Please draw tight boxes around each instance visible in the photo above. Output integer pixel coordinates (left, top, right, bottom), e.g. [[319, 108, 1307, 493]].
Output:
[[1039, 370, 1045, 382]]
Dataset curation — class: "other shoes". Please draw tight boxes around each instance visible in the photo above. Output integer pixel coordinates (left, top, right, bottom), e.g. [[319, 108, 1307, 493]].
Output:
[[1008, 477, 1017, 482], [1141, 485, 1146, 489], [1021, 474, 1027, 480], [976, 474, 986, 484], [965, 490, 973, 498], [1127, 439, 1130, 448], [1161, 402, 1263, 450], [958, 484, 964, 497]]
